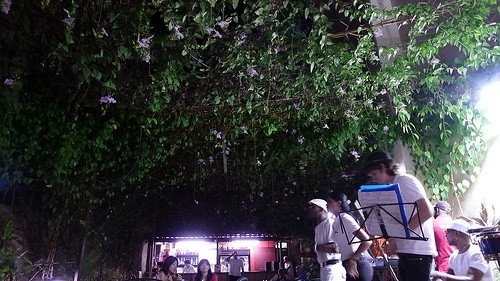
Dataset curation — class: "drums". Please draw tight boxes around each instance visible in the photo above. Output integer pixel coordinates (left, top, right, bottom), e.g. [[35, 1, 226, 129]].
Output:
[[478, 235, 500, 261]]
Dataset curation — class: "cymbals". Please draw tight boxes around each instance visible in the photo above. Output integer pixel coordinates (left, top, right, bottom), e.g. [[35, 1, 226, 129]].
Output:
[[467, 227, 495, 233], [476, 231, 500, 237]]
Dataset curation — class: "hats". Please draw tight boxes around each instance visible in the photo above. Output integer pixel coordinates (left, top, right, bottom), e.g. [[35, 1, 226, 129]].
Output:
[[439, 220, 470, 237], [434, 200, 452, 213], [309, 199, 329, 212], [362, 150, 393, 171]]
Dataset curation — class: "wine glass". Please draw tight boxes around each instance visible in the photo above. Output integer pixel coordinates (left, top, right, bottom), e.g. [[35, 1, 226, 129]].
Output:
[[152, 265, 160, 279]]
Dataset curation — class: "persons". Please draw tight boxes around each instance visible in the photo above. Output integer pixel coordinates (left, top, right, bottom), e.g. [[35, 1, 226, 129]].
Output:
[[294, 265, 308, 281], [425, 222, 493, 281], [431, 201, 459, 281], [226, 251, 244, 281], [155, 256, 185, 281], [326, 191, 373, 281], [368, 150, 439, 281], [195, 259, 218, 281], [182, 260, 197, 273], [308, 198, 346, 281], [283, 256, 294, 281]]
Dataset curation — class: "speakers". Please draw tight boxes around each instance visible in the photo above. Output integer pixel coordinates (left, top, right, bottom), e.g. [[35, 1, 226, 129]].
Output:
[[265, 261, 271, 271], [272, 261, 279, 271]]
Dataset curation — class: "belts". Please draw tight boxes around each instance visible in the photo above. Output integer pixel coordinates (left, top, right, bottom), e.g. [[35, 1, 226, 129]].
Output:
[[321, 259, 338, 268], [399, 254, 432, 262]]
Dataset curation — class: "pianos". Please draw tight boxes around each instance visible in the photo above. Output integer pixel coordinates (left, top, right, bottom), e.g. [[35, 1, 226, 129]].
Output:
[[366, 255, 400, 270]]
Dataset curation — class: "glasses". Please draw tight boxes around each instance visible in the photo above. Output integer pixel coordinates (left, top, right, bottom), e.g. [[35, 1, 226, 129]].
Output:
[[308, 205, 316, 209]]
